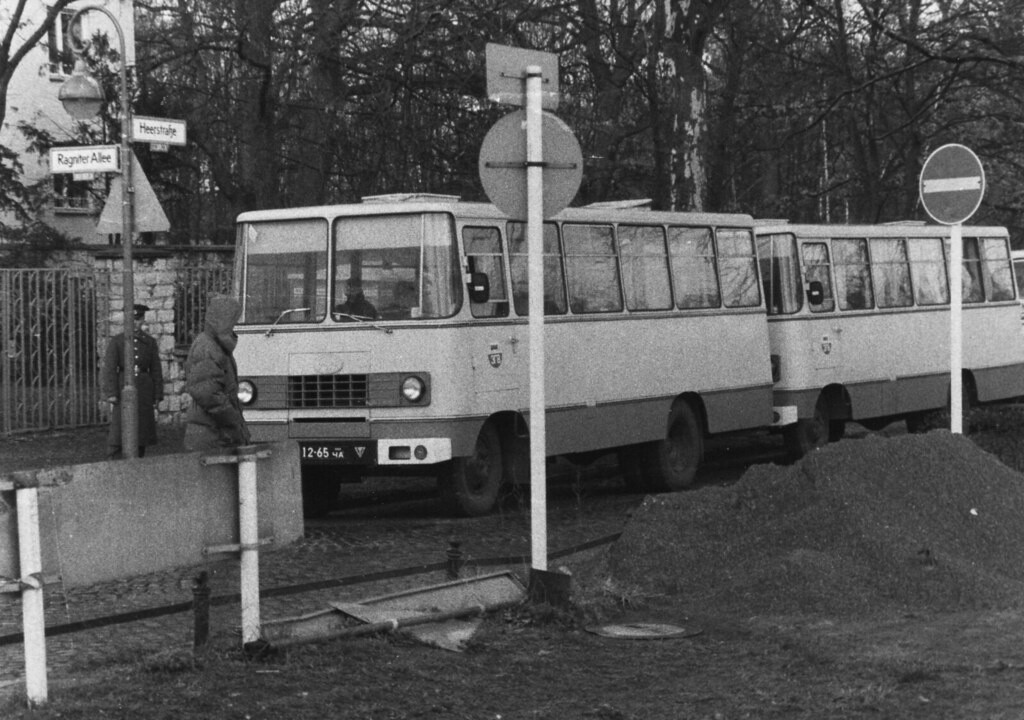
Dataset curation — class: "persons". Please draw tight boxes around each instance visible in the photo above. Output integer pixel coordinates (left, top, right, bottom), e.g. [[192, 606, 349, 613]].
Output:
[[335, 277, 375, 321], [183, 297, 251, 451], [101, 304, 164, 460]]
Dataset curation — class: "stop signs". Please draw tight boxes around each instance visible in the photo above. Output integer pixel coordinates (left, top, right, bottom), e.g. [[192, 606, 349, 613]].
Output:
[[919, 144, 986, 225]]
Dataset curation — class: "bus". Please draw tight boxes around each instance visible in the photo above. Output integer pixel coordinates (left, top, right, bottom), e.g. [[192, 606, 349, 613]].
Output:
[[754, 219, 1024, 458], [229, 197, 775, 514]]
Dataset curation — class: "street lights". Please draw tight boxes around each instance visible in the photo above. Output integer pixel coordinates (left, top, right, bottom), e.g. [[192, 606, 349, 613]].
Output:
[[58, 4, 140, 460]]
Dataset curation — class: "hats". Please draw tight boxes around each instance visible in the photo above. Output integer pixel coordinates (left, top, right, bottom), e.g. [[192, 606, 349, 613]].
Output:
[[121, 304, 149, 319]]
